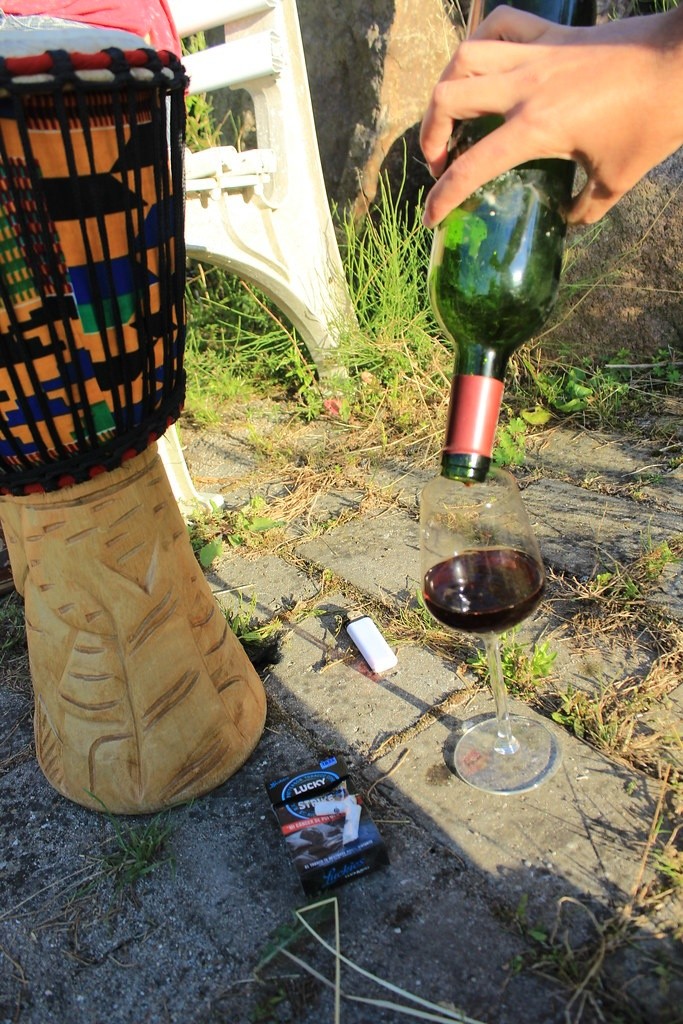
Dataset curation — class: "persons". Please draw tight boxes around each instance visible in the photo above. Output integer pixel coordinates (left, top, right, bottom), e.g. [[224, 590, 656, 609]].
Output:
[[419, 6, 683, 229]]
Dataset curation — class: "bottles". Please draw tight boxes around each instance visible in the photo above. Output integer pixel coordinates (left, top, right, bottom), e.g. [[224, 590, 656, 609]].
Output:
[[428, 0, 598, 484]]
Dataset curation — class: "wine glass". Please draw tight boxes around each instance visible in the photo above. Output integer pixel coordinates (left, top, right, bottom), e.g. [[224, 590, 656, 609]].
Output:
[[420, 468, 563, 794]]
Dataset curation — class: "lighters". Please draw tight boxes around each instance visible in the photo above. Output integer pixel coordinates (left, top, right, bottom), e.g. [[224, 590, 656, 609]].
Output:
[[341, 609, 398, 673]]
